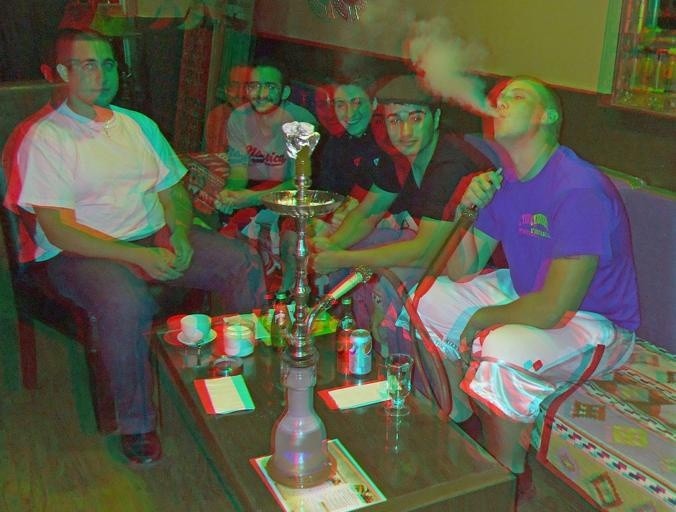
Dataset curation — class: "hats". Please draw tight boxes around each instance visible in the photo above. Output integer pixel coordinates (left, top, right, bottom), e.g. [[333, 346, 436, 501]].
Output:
[[375, 74, 436, 107]]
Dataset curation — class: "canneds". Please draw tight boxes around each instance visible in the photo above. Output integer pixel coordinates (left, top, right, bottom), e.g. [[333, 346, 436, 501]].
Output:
[[337, 329, 352, 374], [349, 329, 372, 376]]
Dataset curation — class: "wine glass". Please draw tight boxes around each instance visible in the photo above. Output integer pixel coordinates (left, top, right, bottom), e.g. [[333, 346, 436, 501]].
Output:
[[384, 354, 415, 417]]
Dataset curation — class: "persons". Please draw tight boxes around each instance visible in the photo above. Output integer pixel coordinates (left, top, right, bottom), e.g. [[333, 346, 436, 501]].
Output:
[[306, 74, 500, 397], [269, 69, 389, 303], [194, 55, 321, 277], [2, 28, 270, 469], [377, 75, 642, 505]]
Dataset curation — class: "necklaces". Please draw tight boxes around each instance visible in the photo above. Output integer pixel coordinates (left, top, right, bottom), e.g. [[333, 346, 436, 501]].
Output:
[[99, 116, 112, 138]]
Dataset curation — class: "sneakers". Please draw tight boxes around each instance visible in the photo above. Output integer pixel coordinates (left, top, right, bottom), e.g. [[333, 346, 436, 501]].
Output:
[[513, 461, 536, 507], [456, 413, 484, 446]]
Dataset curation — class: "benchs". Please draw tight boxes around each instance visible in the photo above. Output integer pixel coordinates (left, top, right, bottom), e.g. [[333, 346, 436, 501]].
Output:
[[174, 149, 232, 216], [530, 162, 676, 510]]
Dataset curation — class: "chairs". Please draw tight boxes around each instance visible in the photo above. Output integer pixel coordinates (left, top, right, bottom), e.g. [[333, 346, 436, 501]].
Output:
[[1, 82, 209, 431]]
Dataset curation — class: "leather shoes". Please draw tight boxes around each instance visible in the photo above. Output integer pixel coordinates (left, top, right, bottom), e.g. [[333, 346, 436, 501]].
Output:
[[121, 431, 162, 464]]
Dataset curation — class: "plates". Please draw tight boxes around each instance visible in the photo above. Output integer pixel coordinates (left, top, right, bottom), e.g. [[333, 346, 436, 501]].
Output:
[[176, 330, 217, 347]]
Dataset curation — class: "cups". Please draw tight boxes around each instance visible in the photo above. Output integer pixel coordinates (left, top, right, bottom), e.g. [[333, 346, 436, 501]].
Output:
[[223, 320, 255, 357], [179, 313, 211, 343]]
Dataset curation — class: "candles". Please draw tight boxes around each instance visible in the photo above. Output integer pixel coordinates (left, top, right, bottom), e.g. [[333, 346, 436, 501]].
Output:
[[279, 120, 322, 204]]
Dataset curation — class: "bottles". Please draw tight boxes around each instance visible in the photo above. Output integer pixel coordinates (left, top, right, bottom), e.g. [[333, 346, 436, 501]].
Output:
[[334, 295, 357, 378], [271, 292, 292, 353]]
